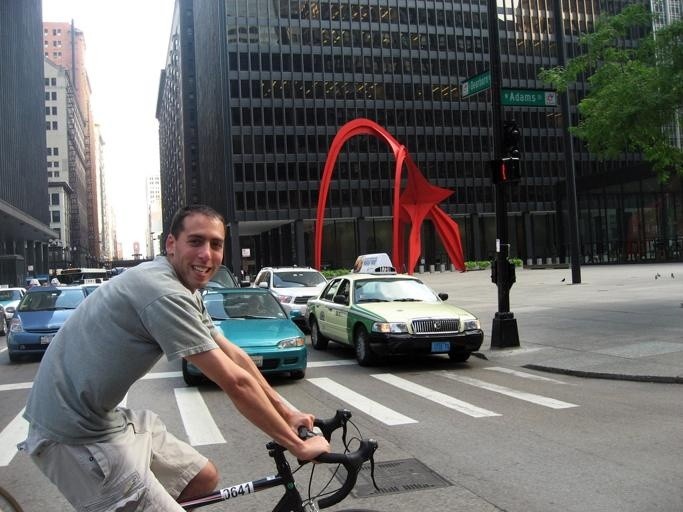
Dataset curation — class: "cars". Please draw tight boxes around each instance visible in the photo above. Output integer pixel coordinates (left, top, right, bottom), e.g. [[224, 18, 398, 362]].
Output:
[[304, 253, 485, 361], [182, 286, 308, 381], [208, 266, 240, 289], [81, 282, 101, 298], [0, 288, 25, 323], [5, 285, 87, 360], [0, 306, 7, 336], [196, 280, 226, 288]]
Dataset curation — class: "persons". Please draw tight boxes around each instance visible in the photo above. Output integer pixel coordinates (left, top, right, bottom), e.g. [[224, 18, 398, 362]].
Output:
[[22, 203, 331, 512]]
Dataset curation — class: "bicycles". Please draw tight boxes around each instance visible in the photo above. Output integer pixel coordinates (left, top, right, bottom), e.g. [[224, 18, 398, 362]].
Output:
[[171, 408, 385, 512]]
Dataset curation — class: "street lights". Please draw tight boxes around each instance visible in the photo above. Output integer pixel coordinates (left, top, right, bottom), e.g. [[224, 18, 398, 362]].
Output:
[[62, 243, 80, 268], [84, 253, 98, 268], [48, 238, 60, 276]]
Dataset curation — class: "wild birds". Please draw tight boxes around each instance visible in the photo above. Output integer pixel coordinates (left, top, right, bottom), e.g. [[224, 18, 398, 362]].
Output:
[[655, 274, 658, 280], [657, 272, 661, 277], [561, 277, 565, 282], [671, 272, 675, 279]]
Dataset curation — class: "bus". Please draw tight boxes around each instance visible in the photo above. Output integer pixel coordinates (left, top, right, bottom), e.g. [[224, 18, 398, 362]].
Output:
[[56, 267, 126, 285]]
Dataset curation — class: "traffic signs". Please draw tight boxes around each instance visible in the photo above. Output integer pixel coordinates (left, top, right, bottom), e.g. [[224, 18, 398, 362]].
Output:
[[499, 86, 556, 110], [460, 71, 493, 96]]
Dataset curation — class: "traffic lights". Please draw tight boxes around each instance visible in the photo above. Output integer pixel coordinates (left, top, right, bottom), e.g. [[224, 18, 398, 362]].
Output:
[[497, 153, 524, 185]]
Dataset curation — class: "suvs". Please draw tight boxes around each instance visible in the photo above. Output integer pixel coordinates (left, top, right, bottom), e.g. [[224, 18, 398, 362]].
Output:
[[251, 263, 332, 334]]
